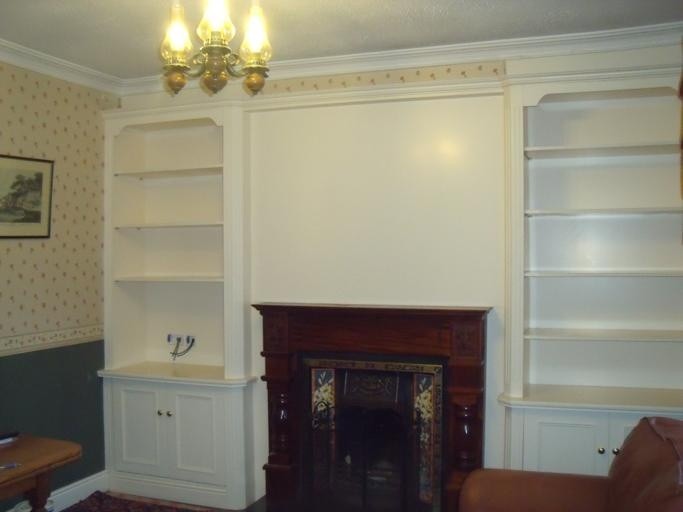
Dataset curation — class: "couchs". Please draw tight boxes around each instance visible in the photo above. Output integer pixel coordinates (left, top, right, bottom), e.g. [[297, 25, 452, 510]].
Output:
[[457, 414, 681, 511]]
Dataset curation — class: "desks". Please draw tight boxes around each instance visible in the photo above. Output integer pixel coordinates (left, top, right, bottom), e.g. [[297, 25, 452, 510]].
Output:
[[0, 431, 83, 512]]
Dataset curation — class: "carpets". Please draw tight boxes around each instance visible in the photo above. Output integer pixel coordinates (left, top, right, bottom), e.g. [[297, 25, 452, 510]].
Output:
[[59, 490, 198, 511]]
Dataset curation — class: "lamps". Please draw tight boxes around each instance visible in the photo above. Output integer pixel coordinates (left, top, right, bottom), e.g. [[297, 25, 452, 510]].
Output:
[[159, 0, 274, 97]]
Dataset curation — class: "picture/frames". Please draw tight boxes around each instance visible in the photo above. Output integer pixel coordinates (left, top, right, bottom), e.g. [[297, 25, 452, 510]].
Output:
[[0, 153, 54, 238]]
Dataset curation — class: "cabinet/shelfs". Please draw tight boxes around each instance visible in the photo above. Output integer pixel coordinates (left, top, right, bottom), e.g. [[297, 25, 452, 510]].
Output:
[[100, 375, 268, 511], [497, 62, 680, 411], [95, 98, 249, 381], [504, 405, 681, 478]]
